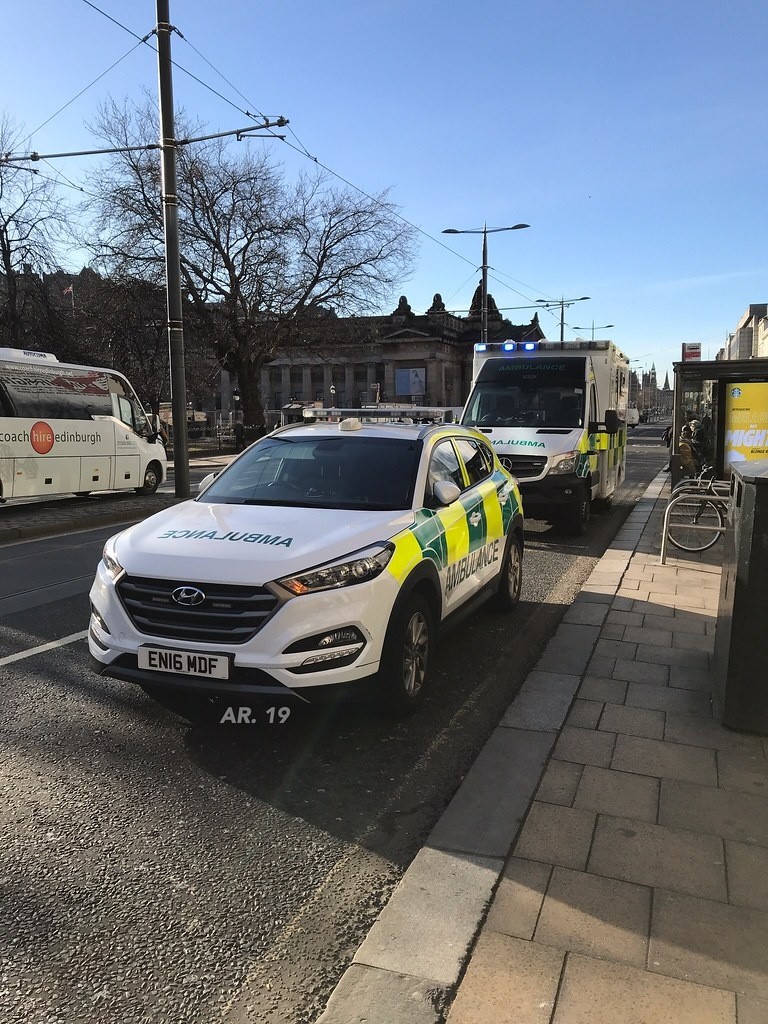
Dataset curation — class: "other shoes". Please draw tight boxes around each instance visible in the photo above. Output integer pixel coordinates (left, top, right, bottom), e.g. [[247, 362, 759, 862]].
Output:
[[663, 468, 671, 472]]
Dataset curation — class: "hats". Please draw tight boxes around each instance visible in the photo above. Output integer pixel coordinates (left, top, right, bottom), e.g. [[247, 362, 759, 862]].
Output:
[[689, 420, 702, 430]]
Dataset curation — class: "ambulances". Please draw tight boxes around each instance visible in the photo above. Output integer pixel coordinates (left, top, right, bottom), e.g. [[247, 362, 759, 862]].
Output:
[[85, 408, 525, 716], [458, 340, 628, 533]]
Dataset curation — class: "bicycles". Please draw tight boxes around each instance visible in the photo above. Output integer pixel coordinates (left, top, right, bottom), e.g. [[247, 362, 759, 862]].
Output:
[[661, 465, 730, 553]]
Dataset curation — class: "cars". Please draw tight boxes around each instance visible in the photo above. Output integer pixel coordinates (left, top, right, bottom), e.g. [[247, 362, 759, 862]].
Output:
[[629, 402, 640, 429]]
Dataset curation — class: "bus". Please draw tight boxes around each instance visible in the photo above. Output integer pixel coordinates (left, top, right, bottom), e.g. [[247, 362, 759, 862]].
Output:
[[0, 345, 169, 504]]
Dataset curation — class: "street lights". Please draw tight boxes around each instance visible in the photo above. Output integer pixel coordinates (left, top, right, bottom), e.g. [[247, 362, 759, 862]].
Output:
[[231, 388, 241, 427], [329, 384, 337, 409], [534, 295, 591, 342], [572, 319, 615, 341], [440, 224, 531, 343]]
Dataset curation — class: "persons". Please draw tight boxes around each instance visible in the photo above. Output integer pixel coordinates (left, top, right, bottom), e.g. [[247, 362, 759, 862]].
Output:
[[234, 419, 244, 449], [689, 420, 707, 479], [663, 404, 711, 473], [274, 420, 281, 430], [153, 419, 168, 444]]
